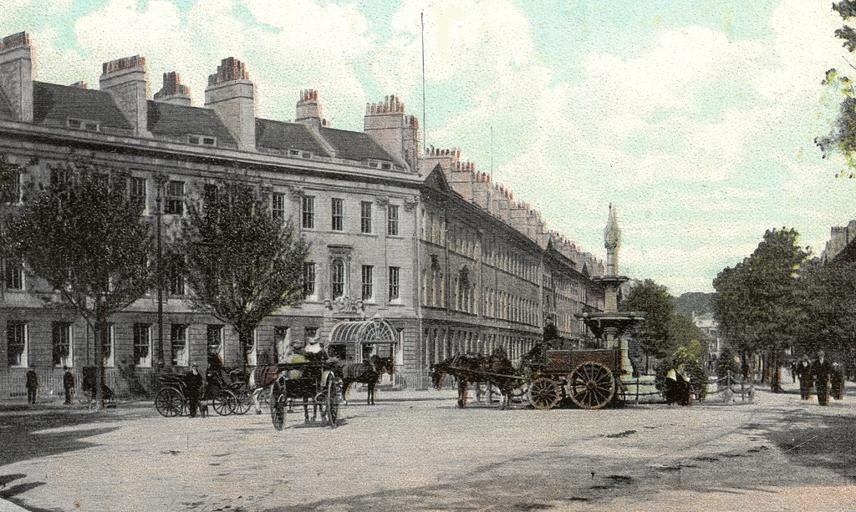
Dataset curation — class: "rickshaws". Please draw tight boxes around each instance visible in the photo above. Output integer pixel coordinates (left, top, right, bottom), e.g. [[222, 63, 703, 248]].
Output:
[[76, 366, 120, 408]]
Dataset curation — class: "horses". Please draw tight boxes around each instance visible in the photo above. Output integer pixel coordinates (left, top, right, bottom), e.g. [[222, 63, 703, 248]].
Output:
[[247, 366, 282, 415], [429, 352, 515, 410], [336, 356, 394, 405]]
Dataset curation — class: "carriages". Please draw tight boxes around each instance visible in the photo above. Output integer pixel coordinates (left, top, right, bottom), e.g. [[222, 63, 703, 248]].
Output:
[[428, 344, 626, 411], [154, 337, 396, 428]]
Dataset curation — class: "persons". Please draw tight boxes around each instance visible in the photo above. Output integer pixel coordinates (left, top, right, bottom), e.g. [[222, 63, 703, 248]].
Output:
[[185, 364, 203, 418], [520, 316, 560, 360], [26, 364, 39, 408], [63, 366, 74, 404], [303, 337, 328, 362], [790, 350, 843, 405]]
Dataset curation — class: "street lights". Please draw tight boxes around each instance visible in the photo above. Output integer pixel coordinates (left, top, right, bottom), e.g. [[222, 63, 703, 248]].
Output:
[[152, 179, 166, 397]]
